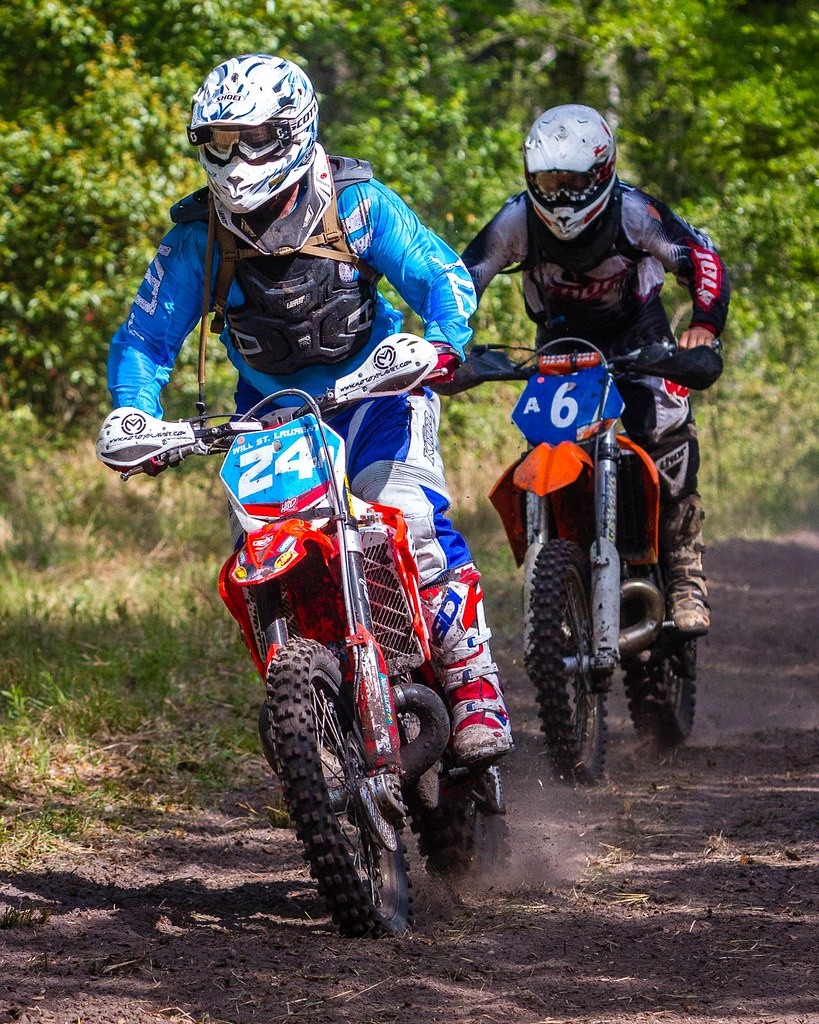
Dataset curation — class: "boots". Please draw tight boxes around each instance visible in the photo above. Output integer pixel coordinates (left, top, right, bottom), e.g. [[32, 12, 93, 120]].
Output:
[[416, 560, 513, 766], [664, 524, 711, 637]]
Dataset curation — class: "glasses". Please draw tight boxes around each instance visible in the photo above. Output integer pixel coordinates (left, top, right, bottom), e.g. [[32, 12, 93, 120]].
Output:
[[532, 169, 597, 199], [187, 97, 319, 166]]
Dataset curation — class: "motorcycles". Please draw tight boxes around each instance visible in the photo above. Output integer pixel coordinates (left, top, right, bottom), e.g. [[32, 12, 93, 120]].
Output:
[[428, 338, 725, 786], [95, 332, 513, 940]]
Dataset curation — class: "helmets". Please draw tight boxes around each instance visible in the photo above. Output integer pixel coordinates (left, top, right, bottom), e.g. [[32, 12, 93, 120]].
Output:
[[521, 103, 617, 242], [190, 51, 320, 215]]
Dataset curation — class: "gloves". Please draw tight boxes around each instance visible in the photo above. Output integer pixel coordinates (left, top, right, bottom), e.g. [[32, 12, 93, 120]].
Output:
[[420, 338, 458, 384]]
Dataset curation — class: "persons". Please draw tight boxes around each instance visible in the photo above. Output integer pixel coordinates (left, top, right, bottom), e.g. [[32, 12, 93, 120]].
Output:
[[461, 104, 730, 634], [107, 55, 514, 766]]
